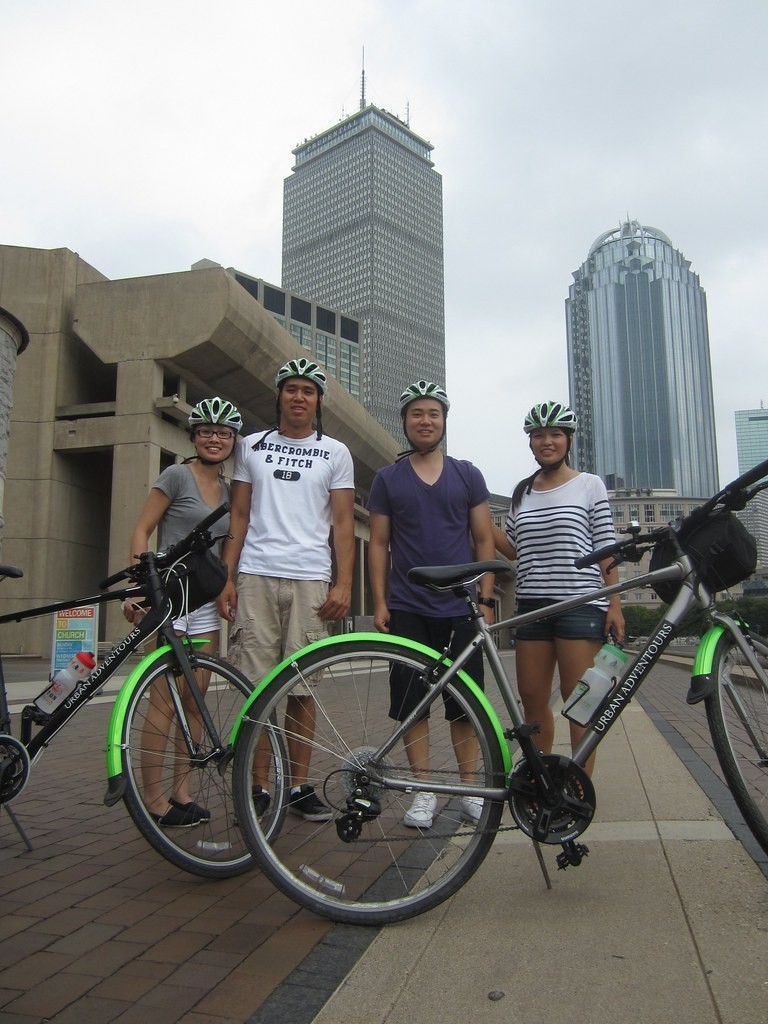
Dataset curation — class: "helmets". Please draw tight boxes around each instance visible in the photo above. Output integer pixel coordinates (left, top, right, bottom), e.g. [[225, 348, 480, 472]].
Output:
[[399, 380, 451, 418], [523, 400, 578, 434], [188, 397, 243, 433], [275, 358, 328, 394]]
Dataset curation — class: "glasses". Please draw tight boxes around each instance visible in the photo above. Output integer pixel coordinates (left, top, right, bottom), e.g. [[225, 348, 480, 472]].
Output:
[[194, 429, 235, 439]]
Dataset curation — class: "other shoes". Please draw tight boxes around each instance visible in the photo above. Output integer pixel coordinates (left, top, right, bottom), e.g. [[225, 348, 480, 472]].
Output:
[[147, 807, 200, 827], [168, 797, 210, 821]]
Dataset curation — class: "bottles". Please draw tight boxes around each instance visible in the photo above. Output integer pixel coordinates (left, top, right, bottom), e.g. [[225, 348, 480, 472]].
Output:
[[562, 642, 629, 725], [33, 651, 98, 714]]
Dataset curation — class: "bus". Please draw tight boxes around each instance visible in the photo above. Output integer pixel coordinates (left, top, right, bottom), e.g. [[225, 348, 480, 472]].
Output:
[[628, 636, 639, 646]]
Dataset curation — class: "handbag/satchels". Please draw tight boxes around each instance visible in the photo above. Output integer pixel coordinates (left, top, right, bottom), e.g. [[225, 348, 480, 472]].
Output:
[[158, 549, 229, 619], [649, 509, 757, 605]]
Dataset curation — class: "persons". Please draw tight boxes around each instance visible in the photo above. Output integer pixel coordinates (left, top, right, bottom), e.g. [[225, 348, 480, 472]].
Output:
[[490, 401, 626, 812], [121, 397, 243, 830], [369, 380, 495, 828], [214, 358, 355, 824]]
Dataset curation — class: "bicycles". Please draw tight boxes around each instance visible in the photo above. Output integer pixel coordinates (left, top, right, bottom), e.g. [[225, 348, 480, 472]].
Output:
[[0, 500, 292, 880], [217, 455, 767, 926]]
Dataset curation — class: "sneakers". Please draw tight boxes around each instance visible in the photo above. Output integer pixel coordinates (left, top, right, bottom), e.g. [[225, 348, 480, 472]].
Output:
[[233, 784, 271, 824], [289, 784, 333, 821], [404, 792, 437, 827], [459, 796, 503, 825]]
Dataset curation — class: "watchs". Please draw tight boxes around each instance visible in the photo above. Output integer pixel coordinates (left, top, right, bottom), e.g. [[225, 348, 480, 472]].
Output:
[[478, 598, 495, 608]]
